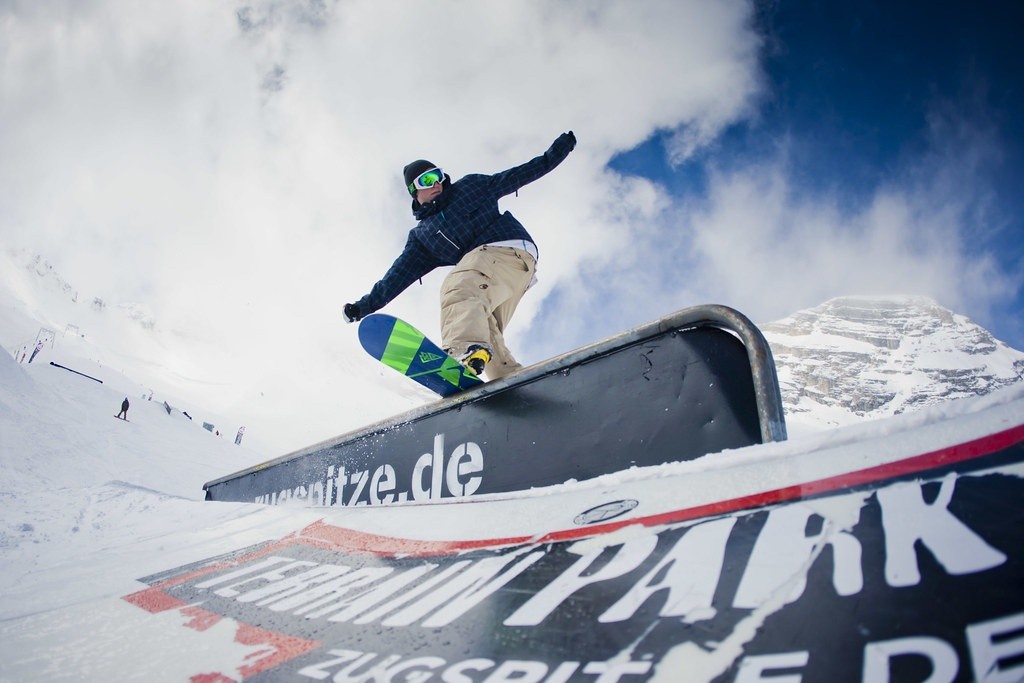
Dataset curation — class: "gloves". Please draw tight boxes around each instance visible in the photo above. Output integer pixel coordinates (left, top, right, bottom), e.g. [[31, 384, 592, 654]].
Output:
[[568, 130, 577, 145], [344, 304, 361, 323]]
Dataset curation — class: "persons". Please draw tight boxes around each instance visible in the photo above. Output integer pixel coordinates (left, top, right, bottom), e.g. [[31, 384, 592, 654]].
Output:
[[341, 131, 577, 380], [118, 397, 129, 420], [216, 430, 219, 435]]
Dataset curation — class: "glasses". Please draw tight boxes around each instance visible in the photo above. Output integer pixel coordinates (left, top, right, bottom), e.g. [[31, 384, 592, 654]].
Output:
[[413, 168, 446, 191]]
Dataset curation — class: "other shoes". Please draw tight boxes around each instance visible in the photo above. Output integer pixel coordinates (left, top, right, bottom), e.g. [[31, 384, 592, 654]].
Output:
[[461, 346, 492, 375]]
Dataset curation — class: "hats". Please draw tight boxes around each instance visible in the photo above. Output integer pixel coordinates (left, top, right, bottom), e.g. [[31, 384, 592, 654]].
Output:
[[403, 159, 439, 196]]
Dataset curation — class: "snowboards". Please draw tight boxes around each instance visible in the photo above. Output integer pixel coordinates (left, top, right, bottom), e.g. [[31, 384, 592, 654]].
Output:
[[114, 415, 130, 422], [356, 312, 487, 399]]
